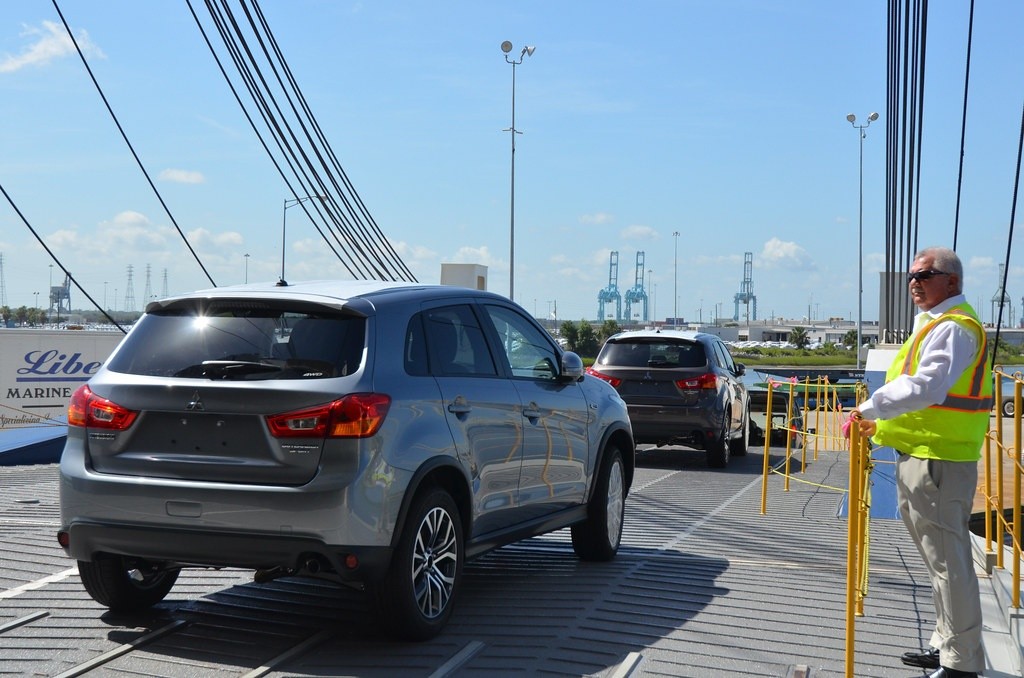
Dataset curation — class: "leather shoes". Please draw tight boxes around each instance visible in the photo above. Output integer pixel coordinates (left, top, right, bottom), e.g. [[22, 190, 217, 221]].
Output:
[[900, 647, 939, 668], [925, 667, 978, 678]]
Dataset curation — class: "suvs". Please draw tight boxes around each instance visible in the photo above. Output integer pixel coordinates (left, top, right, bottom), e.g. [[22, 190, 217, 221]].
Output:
[[56, 277, 636, 641], [584, 327, 754, 467]]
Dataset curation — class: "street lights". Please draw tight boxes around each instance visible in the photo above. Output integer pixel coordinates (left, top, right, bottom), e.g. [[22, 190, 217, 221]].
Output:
[[670, 231, 681, 329], [501, 40, 536, 373], [33, 292, 40, 324], [845, 110, 880, 379], [243, 252, 249, 283], [48, 265, 54, 327], [277, 194, 328, 277]]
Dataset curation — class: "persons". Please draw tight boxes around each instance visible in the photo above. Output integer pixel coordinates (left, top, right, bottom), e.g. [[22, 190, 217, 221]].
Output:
[[849, 245, 995, 678]]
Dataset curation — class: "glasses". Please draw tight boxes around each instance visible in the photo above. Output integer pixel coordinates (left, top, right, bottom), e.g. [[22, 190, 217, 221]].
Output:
[[907, 270, 948, 284]]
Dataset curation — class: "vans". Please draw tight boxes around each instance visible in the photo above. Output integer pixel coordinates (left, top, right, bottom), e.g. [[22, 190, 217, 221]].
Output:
[[746, 385, 816, 450]]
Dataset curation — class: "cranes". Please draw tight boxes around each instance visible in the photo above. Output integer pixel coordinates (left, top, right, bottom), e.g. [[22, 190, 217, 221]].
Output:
[[598, 250, 622, 324], [734, 252, 758, 321], [625, 249, 650, 322]]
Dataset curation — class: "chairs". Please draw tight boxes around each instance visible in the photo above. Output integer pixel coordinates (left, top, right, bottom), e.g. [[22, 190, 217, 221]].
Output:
[[679, 348, 702, 363], [624, 348, 649, 366], [411, 318, 459, 375]]
